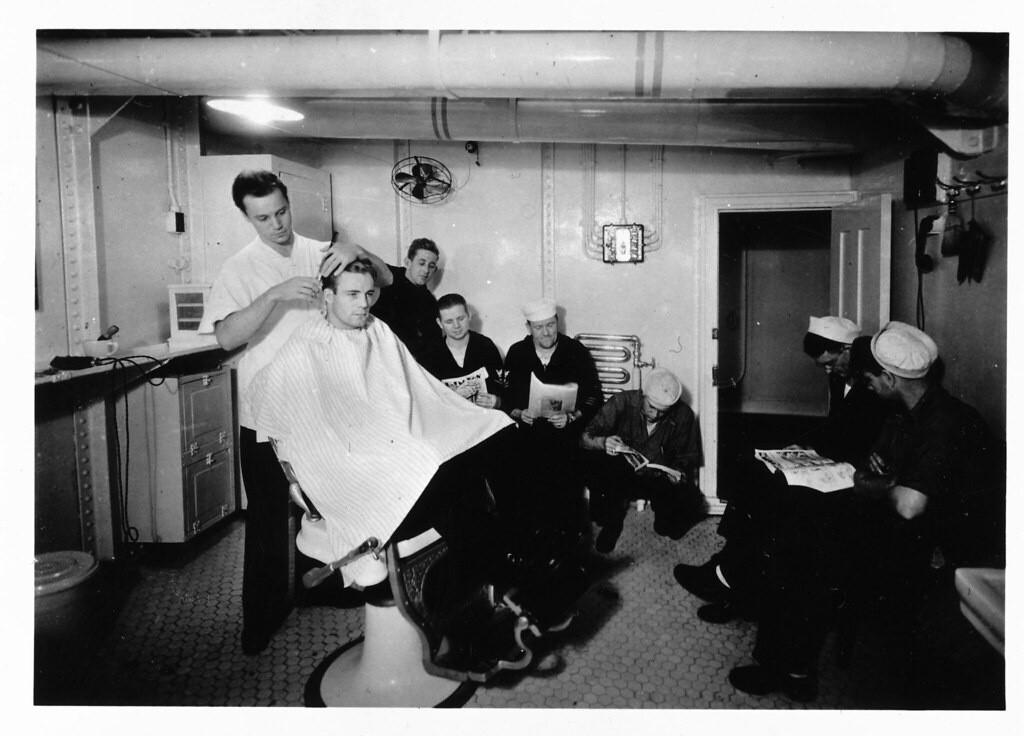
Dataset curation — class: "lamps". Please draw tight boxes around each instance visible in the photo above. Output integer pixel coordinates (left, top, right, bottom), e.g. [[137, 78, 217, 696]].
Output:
[[202, 95, 306, 121]]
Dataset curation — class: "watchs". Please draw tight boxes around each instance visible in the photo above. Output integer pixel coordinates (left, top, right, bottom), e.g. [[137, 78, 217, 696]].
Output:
[[569, 411, 577, 422]]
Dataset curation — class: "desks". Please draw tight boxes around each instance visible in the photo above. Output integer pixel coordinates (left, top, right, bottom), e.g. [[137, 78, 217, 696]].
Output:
[[35, 341, 221, 561]]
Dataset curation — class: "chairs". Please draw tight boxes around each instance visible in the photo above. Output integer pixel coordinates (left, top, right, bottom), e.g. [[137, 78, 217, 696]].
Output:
[[267, 434, 573, 708]]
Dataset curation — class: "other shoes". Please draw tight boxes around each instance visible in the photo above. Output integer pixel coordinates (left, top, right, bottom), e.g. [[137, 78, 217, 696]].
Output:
[[241, 623, 273, 658], [729, 663, 820, 704], [595, 519, 624, 555], [673, 553, 736, 606], [652, 521, 684, 541], [698, 602, 757, 624], [299, 585, 367, 609]]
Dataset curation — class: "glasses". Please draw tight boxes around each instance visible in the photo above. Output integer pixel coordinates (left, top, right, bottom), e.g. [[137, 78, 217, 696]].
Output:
[[815, 358, 838, 370]]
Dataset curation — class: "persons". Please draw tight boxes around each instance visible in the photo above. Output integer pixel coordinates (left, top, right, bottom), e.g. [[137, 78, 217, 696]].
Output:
[[672, 312, 983, 711], [198, 165, 711, 672]]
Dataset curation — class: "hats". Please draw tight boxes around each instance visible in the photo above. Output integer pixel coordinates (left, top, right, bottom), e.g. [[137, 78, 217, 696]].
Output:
[[807, 316, 863, 345], [641, 367, 683, 413], [521, 298, 557, 322], [883, 320, 939, 366], [870, 326, 928, 379]]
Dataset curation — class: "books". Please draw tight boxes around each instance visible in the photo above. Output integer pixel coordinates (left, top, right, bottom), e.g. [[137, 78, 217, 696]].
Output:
[[755, 449, 857, 493], [615, 443, 681, 479]]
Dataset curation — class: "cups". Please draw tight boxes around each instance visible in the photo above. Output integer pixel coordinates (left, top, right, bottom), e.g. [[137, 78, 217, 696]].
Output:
[[83, 340, 118, 359]]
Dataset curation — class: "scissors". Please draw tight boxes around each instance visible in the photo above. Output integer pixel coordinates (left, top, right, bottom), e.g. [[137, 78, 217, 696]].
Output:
[[311, 272, 323, 299]]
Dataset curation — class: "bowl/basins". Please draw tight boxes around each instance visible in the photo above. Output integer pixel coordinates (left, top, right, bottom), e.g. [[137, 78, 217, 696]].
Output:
[[35, 551, 99, 637]]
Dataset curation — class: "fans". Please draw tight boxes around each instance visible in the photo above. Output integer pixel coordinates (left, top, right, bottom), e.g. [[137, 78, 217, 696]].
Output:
[[391, 156, 452, 204]]
